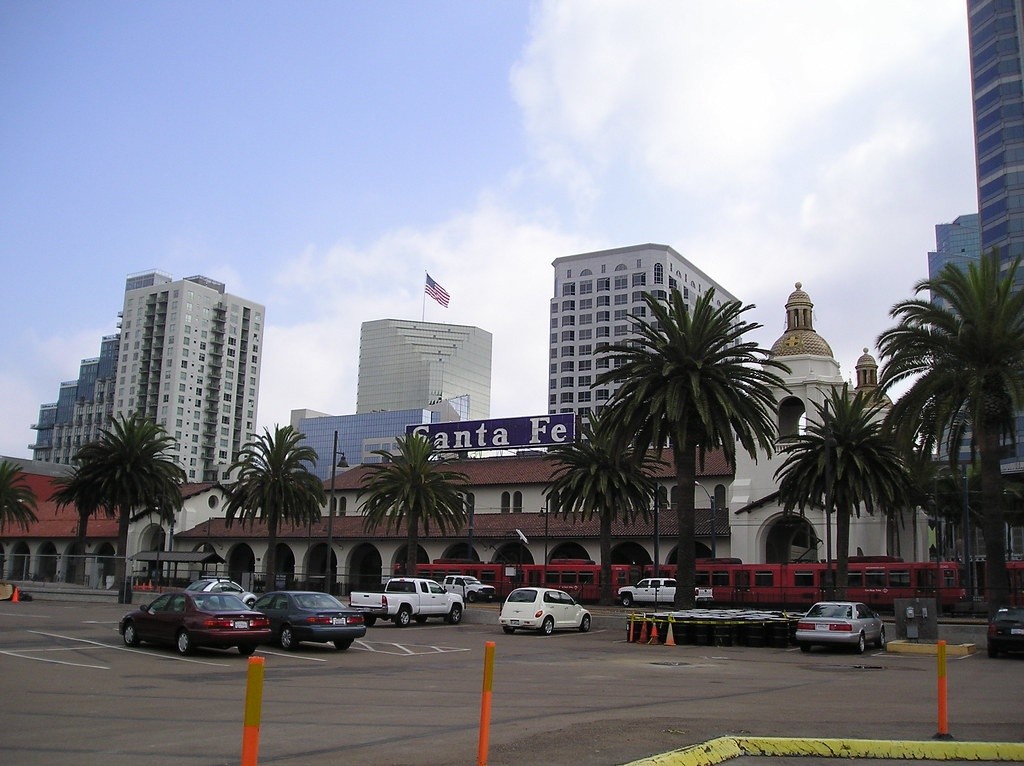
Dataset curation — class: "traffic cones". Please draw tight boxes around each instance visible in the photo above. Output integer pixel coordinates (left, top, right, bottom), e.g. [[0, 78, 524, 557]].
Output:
[[155, 584, 158, 592], [142, 583, 146, 591], [664, 622, 677, 646], [650, 623, 662, 645], [12, 588, 19, 603], [148, 579, 152, 589], [638, 621, 648, 644]]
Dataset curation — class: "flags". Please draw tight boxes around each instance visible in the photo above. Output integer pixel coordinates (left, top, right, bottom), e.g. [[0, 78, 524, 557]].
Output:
[[424, 272, 450, 308]]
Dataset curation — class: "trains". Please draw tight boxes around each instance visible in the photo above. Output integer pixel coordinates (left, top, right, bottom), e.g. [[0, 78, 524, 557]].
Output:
[[394, 558, 1024, 614]]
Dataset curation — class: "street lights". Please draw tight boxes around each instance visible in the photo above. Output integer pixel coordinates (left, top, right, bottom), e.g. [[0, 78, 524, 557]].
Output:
[[654, 480, 670, 577], [456, 495, 475, 559], [539, 498, 548, 588], [323, 430, 350, 594], [936, 464, 975, 613], [695, 481, 716, 559], [153, 494, 165, 593]]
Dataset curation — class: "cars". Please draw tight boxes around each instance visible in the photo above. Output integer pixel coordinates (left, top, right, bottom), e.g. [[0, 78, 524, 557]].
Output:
[[987, 607, 1024, 658], [795, 602, 886, 654], [251, 591, 366, 650], [119, 591, 272, 657], [184, 576, 257, 609], [500, 587, 592, 636]]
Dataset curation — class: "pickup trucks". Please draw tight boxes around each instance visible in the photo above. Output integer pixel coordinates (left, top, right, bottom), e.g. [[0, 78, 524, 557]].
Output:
[[618, 577, 713, 608], [438, 575, 496, 603], [349, 578, 464, 627]]
